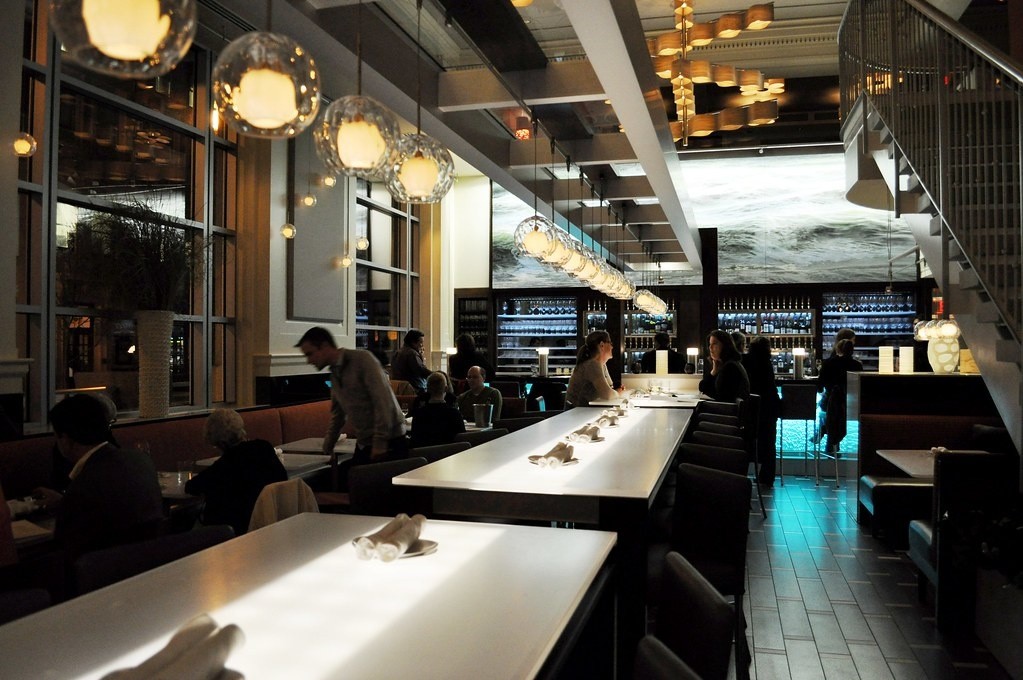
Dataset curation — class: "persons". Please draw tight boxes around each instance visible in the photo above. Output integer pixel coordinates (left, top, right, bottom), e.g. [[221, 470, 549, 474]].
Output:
[[186, 409, 289, 528], [294, 327, 408, 475], [699, 331, 780, 487], [632, 333, 696, 374], [554, 339, 575, 365], [42, 392, 122, 496], [564, 331, 614, 414], [518, 337, 541, 372], [46, 395, 172, 550], [810, 329, 864, 459], [392, 329, 503, 446]]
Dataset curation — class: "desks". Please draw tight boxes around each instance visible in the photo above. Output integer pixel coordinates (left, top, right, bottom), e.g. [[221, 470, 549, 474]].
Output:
[[586, 387, 706, 408], [4, 514, 618, 677], [875, 447, 987, 479], [11, 409, 488, 546], [391, 403, 692, 503]]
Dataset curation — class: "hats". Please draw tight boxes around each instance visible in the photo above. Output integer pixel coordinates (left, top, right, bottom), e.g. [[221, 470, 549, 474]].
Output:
[[746, 336, 770, 353]]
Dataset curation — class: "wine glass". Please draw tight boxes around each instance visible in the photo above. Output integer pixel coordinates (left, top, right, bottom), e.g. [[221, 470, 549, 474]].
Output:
[[824, 294, 914, 312], [822, 318, 912, 334], [497, 296, 577, 315], [500, 320, 577, 336], [531, 363, 538, 377]]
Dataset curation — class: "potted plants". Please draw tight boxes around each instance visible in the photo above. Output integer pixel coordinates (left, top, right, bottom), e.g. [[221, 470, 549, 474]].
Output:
[[60, 206, 226, 417]]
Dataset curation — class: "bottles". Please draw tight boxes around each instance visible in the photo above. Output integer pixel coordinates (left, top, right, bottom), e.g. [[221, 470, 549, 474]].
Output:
[[588, 313, 673, 334], [770, 356, 812, 374], [718, 312, 812, 334]]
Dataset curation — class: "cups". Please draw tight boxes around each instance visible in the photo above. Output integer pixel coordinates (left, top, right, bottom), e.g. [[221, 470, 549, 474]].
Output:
[[566, 340, 577, 349], [177, 461, 193, 484], [504, 350, 536, 358], [502, 337, 521, 347], [402, 402, 408, 417], [823, 352, 868, 360], [822, 336, 836, 347]]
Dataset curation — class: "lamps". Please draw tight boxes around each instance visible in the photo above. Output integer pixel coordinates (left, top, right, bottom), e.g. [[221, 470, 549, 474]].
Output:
[[793, 346, 805, 356], [316, 0, 401, 180], [381, 0, 453, 205], [204, 0, 323, 139], [338, 253, 354, 271], [355, 180, 369, 252], [512, 1, 787, 320], [322, 170, 338, 191], [280, 212, 296, 241], [686, 347, 699, 357], [538, 347, 550, 356], [914, 306, 962, 341], [300, 120, 316, 208], [44, 1, 198, 79], [11, 128, 39, 159]]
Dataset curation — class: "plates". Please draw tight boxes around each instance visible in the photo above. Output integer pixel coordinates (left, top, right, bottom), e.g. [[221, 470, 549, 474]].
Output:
[[565, 436, 605, 442], [609, 424, 618, 427], [352, 536, 438, 558], [528, 455, 577, 465], [101, 666, 244, 679]]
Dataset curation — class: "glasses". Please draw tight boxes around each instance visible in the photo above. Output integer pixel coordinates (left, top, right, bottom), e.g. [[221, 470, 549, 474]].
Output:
[[306, 350, 318, 356]]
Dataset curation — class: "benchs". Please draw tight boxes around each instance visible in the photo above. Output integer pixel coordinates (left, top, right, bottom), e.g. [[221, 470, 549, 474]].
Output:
[[0, 393, 419, 498], [858, 412, 1023, 679]]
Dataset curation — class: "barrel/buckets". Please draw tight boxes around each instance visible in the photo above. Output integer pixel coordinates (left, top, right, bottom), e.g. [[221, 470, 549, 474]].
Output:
[[473, 404, 494, 427]]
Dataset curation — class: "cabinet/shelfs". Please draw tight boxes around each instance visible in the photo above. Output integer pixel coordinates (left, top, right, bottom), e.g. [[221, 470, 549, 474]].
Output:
[[719, 308, 815, 338], [496, 315, 577, 377], [822, 311, 915, 361]]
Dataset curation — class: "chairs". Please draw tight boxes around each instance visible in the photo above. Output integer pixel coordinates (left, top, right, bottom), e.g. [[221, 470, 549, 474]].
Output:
[[245, 478, 319, 532], [73, 526, 234, 593]]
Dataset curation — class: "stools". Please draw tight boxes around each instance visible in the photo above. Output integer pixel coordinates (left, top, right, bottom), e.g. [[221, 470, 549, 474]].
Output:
[[561, 384, 844, 680], [348, 406, 557, 519]]
